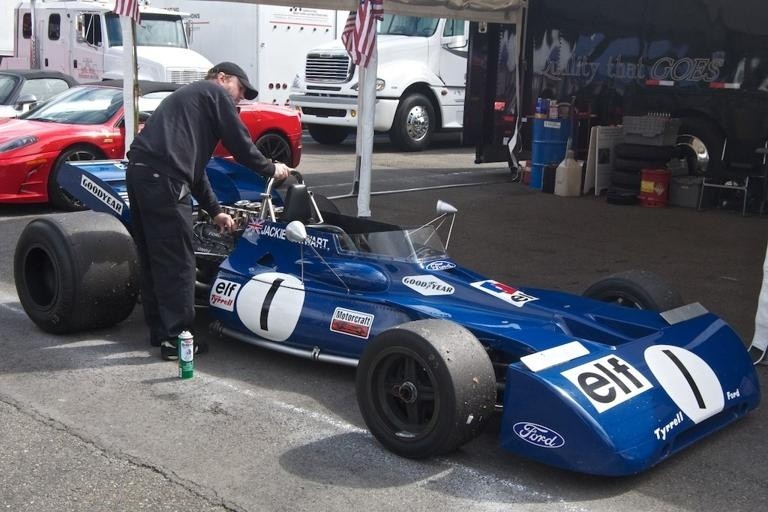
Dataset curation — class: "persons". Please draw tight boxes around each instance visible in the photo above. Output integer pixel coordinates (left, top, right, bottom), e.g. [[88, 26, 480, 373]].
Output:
[[125, 61, 290, 362]]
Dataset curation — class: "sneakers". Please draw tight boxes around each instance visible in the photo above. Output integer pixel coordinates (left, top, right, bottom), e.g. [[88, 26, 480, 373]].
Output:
[[159, 337, 210, 361]]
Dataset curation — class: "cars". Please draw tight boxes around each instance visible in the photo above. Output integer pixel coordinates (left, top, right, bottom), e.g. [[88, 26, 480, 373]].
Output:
[[0, 69, 79, 118]]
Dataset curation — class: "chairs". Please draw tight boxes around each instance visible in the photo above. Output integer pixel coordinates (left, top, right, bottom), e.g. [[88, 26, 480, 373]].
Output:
[[698, 136, 766, 216], [283, 184, 311, 223]]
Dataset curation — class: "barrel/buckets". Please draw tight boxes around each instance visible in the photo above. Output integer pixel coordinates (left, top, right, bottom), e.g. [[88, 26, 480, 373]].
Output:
[[529, 117, 571, 187], [639, 168, 672, 207]]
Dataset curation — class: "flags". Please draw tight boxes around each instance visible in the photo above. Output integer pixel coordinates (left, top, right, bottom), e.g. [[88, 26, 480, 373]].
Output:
[[113, 0, 140, 24], [341, 0, 382, 68], [245, 217, 266, 234]]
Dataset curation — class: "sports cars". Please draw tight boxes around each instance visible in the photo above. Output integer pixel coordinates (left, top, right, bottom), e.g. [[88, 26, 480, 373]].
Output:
[[0, 79, 302, 211]]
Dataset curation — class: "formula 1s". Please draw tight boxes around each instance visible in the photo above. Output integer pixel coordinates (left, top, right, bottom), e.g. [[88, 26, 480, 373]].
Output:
[[11, 154, 762, 477]]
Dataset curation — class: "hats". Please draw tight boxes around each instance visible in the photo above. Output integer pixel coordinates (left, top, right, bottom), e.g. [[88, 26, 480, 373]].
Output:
[[208, 61, 259, 102]]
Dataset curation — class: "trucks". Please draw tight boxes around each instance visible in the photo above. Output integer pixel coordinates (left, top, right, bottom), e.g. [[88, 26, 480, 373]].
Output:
[[0, 0, 216, 86], [289, 11, 471, 151]]
